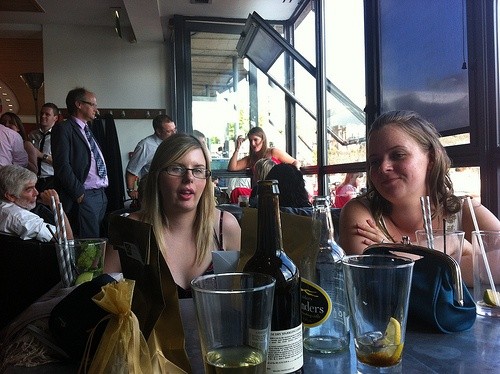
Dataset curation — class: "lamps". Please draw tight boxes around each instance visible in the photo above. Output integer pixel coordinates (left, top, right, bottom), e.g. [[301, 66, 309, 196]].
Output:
[[19, 71, 44, 127]]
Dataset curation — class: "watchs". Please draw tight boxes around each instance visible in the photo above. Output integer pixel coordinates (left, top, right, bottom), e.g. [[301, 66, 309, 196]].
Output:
[[43, 154, 48, 161]]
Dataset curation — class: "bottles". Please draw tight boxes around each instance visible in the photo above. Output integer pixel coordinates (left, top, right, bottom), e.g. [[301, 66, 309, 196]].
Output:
[[240, 179, 305, 374], [298, 195, 353, 353]]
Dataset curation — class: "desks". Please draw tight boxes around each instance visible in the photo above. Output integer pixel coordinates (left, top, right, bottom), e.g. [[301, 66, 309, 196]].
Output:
[[178, 282, 500, 374]]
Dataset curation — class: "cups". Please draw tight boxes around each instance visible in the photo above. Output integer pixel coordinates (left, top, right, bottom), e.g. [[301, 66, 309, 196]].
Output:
[[238, 194, 249, 206], [54, 236, 108, 294], [190, 272, 276, 374], [338, 254, 415, 374], [470, 230, 500, 318], [414, 229, 466, 263]]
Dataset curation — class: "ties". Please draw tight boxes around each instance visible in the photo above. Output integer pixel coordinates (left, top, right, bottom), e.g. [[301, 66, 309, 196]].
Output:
[[84, 125, 107, 179], [37, 131, 51, 176]]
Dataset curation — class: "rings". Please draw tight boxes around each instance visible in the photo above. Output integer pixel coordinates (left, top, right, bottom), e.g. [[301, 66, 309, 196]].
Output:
[[381, 238, 386, 243]]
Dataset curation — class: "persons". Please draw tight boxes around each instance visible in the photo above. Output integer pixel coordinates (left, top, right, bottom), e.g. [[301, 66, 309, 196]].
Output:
[[28, 103, 62, 204], [228, 126, 312, 207], [338, 110, 500, 288], [104, 134, 241, 299], [193, 130, 206, 148], [335, 172, 365, 208], [51, 88, 109, 239], [125, 114, 177, 199], [0, 165, 75, 242], [0, 99, 37, 169]]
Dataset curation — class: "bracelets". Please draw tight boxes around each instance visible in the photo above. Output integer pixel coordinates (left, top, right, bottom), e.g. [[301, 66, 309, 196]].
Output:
[[127, 189, 132, 193]]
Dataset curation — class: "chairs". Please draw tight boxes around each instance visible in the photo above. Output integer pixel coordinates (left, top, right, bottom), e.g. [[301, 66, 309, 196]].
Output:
[[0, 234, 61, 328]]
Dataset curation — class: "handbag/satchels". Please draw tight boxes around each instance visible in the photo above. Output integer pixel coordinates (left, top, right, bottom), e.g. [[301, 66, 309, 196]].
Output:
[[48, 212, 192, 374], [363, 234, 476, 334]]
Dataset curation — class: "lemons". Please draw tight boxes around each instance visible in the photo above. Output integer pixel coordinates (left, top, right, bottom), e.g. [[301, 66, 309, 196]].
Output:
[[75, 273, 93, 285], [377, 317, 404, 361], [483, 289, 500, 306]]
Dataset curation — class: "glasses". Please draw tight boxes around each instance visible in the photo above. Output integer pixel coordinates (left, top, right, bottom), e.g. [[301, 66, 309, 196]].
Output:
[[166, 164, 212, 179], [78, 99, 99, 108]]
